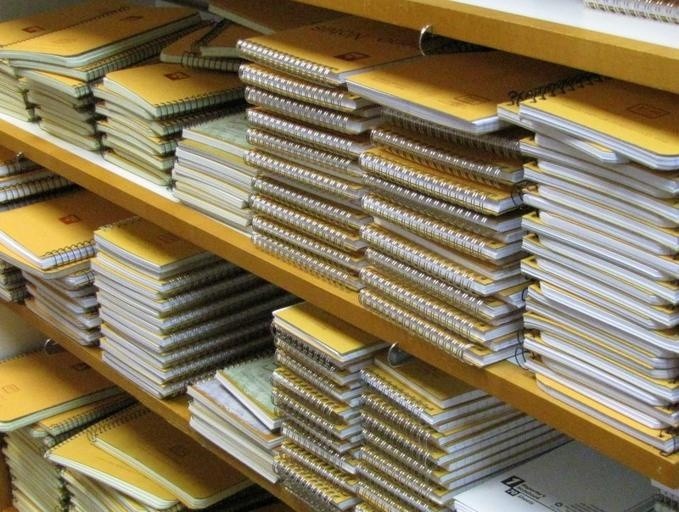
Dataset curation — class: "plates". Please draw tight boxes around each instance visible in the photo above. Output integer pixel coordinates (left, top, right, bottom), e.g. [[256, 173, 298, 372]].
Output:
[[2, 0, 679, 510]]
